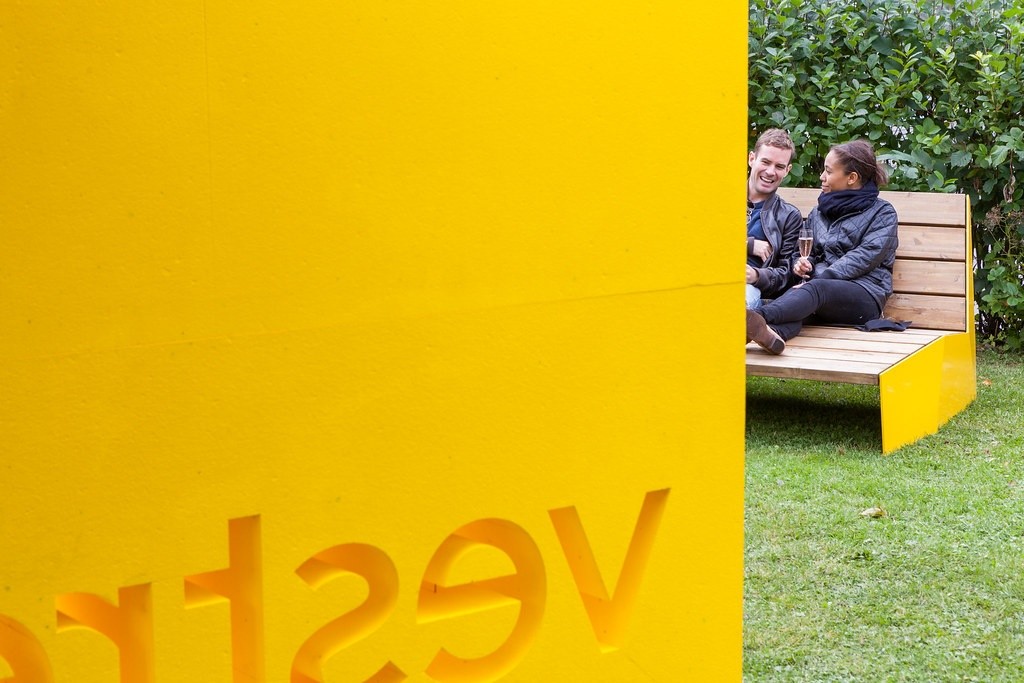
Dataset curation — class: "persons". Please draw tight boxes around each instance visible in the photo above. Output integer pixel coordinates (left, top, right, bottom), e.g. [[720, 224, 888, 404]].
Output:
[[747, 128, 898, 355]]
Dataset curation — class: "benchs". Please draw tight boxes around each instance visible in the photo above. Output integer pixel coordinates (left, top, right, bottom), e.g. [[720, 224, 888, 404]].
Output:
[[746, 185, 976, 455]]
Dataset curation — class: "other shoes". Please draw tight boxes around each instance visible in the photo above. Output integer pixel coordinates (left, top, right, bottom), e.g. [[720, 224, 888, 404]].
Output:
[[746, 308, 785, 356]]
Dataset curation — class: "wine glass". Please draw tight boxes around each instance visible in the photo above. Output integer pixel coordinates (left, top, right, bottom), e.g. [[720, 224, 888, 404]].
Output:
[[799, 230, 813, 278]]
